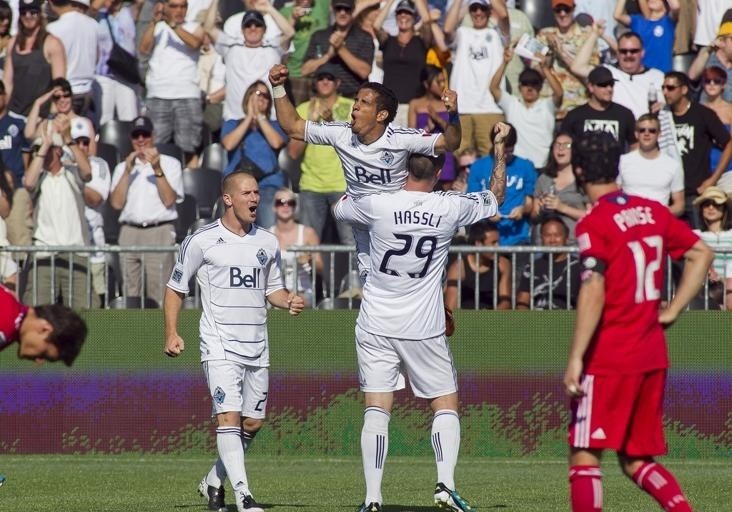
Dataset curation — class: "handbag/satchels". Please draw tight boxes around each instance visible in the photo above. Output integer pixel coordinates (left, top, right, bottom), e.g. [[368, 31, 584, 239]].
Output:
[[233, 156, 264, 182], [106, 44, 140, 82]]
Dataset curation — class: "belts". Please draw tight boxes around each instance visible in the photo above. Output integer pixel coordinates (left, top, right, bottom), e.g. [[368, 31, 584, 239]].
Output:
[[123, 221, 172, 227]]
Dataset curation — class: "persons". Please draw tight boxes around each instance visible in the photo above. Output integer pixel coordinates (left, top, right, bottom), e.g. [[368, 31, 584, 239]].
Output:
[[562, 130, 715, 510], [1, 0, 732, 310], [1, 285, 89, 368], [163, 170, 304, 512], [332, 123, 509, 511]]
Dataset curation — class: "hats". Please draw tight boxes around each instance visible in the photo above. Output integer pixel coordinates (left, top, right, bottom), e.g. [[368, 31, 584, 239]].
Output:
[[692, 186, 728, 207], [19, 0, 40, 10], [34, 117, 153, 146]]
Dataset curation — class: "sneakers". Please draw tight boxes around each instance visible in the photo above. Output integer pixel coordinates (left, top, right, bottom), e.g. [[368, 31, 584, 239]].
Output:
[[197, 474, 228, 512], [434, 483, 477, 512], [236, 492, 264, 512], [356, 503, 383, 512]]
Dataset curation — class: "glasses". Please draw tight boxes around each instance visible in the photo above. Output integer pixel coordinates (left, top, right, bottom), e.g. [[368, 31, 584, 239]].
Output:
[[20, 9, 38, 13], [53, 94, 70, 99], [275, 199, 296, 207], [553, 49, 721, 148], [255, 90, 269, 99]]
